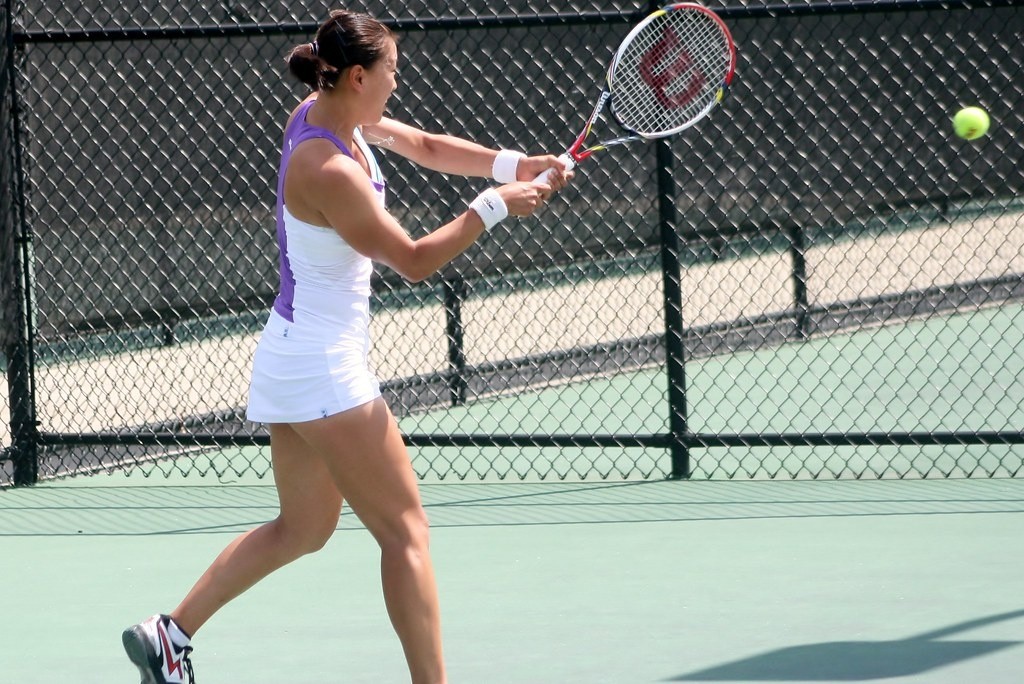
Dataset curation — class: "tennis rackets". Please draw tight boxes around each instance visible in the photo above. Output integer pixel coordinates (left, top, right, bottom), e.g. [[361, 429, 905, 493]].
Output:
[[530, 3, 737, 188]]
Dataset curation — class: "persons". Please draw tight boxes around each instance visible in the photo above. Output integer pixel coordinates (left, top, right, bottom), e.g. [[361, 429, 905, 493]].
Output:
[[122, 8, 576, 684]]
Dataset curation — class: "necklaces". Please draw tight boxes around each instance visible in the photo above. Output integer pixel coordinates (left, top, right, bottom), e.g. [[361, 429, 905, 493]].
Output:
[[329, 123, 395, 146]]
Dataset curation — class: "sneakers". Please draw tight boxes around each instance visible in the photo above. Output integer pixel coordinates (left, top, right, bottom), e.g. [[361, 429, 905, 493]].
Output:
[[122, 614, 195, 684]]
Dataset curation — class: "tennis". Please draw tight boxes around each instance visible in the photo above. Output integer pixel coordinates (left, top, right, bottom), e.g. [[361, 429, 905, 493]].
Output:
[[951, 106, 990, 141]]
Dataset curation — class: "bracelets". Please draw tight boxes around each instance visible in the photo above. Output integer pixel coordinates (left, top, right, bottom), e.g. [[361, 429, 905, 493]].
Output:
[[470, 188, 509, 230], [492, 149, 528, 184]]
